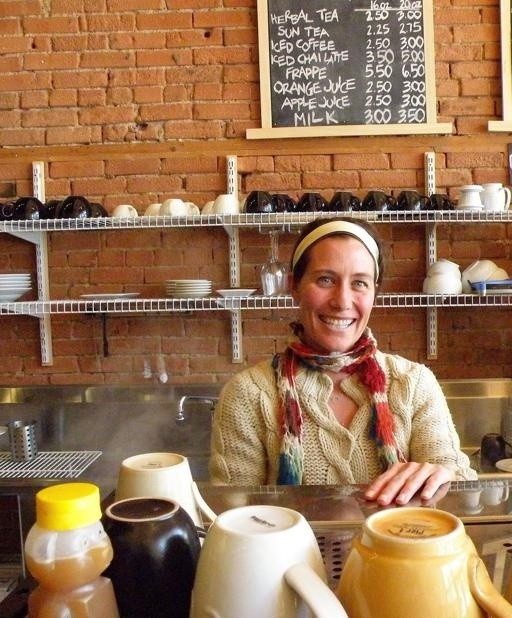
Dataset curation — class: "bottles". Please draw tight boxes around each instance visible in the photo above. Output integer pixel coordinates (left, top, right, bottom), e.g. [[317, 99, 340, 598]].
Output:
[[23, 482, 119, 618], [257, 259, 289, 297]]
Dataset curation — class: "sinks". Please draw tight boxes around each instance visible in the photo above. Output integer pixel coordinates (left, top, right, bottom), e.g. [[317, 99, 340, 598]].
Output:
[[93, 399, 215, 482]]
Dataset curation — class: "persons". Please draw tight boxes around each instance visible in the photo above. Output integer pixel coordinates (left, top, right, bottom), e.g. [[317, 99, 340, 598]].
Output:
[[208, 215, 476, 507]]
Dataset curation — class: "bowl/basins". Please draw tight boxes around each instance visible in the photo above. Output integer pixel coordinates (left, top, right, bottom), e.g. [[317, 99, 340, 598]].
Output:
[[422, 259, 508, 293]]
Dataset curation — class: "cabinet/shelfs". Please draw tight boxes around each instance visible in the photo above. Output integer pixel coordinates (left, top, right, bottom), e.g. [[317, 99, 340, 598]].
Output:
[[0, 207, 512, 320]]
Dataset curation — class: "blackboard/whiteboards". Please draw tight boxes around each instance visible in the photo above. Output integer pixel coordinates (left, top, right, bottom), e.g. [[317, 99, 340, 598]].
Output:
[[245, 0, 453, 140]]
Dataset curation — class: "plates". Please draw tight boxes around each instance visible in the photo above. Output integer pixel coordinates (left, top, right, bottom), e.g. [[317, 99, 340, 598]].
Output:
[[0, 273, 35, 300], [162, 278, 258, 297], [81, 292, 139, 298]]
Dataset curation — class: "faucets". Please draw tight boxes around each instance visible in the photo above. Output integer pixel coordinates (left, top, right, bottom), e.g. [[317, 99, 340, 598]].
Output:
[[174, 394, 217, 426]]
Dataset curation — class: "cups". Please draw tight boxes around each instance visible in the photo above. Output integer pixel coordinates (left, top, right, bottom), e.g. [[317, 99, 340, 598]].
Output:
[[458, 478, 512, 517], [114, 451, 220, 547], [102, 496, 202, 615], [336, 506, 512, 618], [3, 183, 512, 218], [188, 504, 347, 617]]
